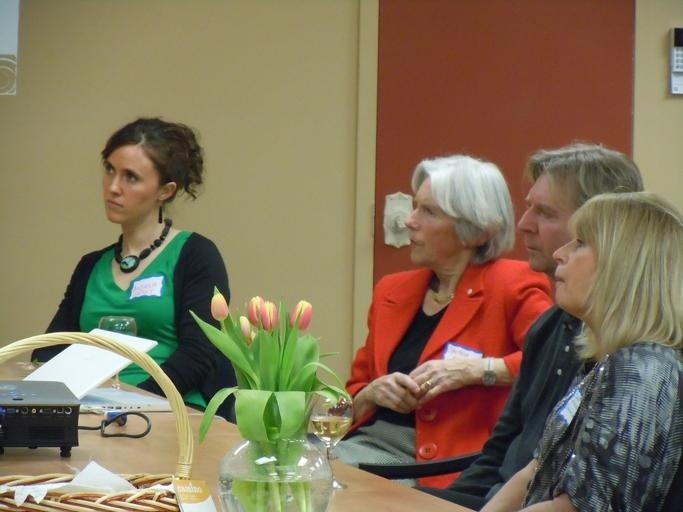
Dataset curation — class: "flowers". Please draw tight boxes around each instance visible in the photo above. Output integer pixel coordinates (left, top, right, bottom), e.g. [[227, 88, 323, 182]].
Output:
[[188, 284, 351, 512]]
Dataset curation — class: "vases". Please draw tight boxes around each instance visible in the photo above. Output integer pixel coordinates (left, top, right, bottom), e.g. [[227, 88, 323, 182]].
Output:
[[217, 433, 334, 512]]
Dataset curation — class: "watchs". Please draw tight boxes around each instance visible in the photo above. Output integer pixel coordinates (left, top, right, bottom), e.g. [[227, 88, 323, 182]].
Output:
[[481, 357, 498, 388]]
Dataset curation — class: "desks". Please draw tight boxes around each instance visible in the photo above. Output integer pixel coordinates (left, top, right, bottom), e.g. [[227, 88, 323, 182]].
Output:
[[0, 362, 475, 512]]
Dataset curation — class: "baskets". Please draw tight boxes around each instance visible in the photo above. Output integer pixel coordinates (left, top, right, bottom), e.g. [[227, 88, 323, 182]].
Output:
[[0, 330, 195, 511]]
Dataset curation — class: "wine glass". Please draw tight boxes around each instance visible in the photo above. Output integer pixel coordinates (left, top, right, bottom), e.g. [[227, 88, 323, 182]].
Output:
[[98, 314, 138, 393], [310, 389, 354, 492]]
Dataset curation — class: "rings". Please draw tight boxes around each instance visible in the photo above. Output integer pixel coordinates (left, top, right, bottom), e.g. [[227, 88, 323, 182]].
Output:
[[425, 381, 433, 389]]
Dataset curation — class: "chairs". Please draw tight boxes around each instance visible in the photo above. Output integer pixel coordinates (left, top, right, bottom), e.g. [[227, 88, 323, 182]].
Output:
[[357, 451, 484, 479]]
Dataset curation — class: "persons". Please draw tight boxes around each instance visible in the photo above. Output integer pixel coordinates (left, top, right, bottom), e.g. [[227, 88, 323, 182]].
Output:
[[115, 219, 172, 273], [478, 190, 683, 512], [333, 154, 558, 488], [411, 142, 645, 512], [31, 119, 230, 416]]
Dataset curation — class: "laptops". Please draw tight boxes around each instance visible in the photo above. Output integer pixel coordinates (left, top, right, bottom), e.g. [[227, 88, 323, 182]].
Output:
[[18, 328, 181, 414]]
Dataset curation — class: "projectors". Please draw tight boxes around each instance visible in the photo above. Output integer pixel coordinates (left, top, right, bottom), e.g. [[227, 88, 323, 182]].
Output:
[[0, 378, 81, 457]]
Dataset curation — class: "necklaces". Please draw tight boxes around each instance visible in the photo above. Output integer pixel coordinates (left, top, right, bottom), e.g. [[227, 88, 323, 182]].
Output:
[[432, 292, 454, 304]]
